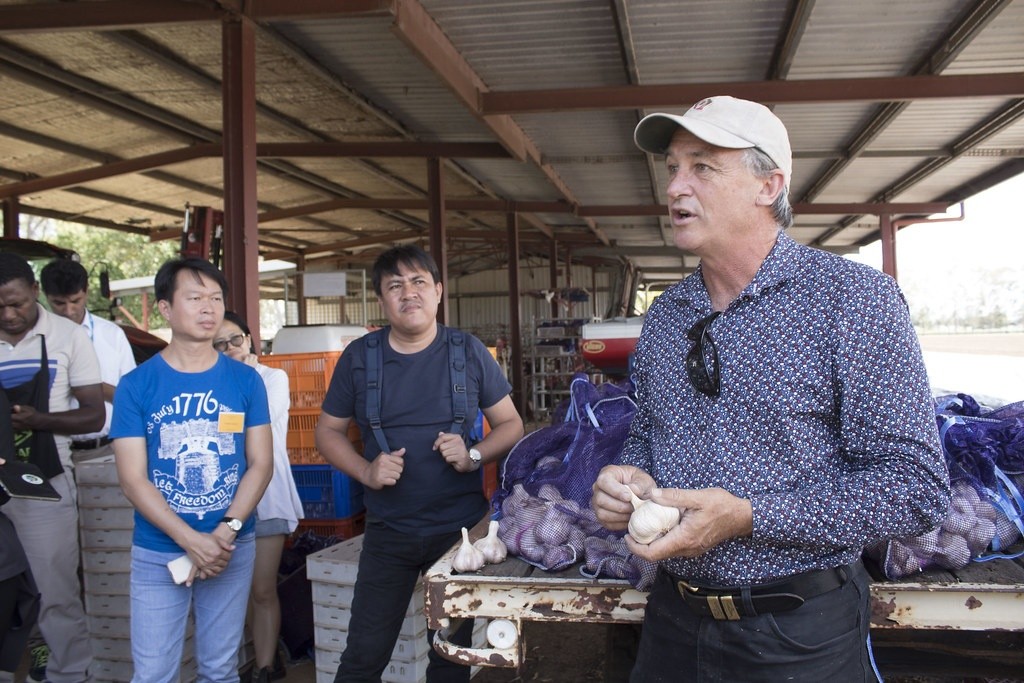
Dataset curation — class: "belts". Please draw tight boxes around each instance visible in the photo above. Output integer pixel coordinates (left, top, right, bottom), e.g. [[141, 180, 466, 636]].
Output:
[[657, 545, 864, 619]]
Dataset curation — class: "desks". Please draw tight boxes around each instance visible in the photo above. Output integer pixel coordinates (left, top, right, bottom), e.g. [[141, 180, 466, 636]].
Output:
[[423, 508, 1023, 683]]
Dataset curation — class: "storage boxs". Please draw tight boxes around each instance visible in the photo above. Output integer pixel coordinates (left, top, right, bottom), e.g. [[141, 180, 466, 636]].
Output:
[[306, 533, 489, 683], [285, 512, 368, 549], [75, 454, 257, 683], [537, 328, 564, 336], [257, 350, 365, 464], [535, 346, 563, 355], [289, 463, 367, 520]]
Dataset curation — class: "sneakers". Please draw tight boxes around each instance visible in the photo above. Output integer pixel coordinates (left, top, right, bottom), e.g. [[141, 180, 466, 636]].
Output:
[[26, 645, 53, 683]]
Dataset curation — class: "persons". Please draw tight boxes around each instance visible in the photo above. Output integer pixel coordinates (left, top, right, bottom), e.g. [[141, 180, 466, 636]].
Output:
[[1, 255, 106, 683], [107, 256, 275, 683], [592, 96, 951, 683], [214, 312, 305, 683], [312, 247, 523, 683], [40, 259, 138, 462]]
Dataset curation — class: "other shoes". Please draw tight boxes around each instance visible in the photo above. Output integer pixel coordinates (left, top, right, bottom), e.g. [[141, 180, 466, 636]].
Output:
[[241, 655, 287, 683]]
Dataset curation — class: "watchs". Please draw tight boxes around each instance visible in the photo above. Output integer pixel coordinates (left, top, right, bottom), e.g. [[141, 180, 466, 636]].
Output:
[[220, 517, 243, 539], [468, 449, 481, 471]]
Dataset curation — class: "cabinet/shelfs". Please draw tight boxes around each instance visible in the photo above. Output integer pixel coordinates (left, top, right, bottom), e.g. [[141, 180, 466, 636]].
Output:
[[530, 314, 602, 430]]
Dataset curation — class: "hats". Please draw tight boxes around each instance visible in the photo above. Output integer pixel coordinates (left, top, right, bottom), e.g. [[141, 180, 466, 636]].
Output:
[[633, 95, 795, 168]]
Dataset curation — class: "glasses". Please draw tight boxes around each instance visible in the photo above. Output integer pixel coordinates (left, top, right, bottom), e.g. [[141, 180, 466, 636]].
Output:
[[686, 307, 723, 402], [213, 332, 248, 354]]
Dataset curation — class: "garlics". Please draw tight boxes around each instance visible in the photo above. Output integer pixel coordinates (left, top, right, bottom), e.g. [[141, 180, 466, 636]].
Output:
[[864, 474, 1024, 581], [452, 483, 680, 591]]
